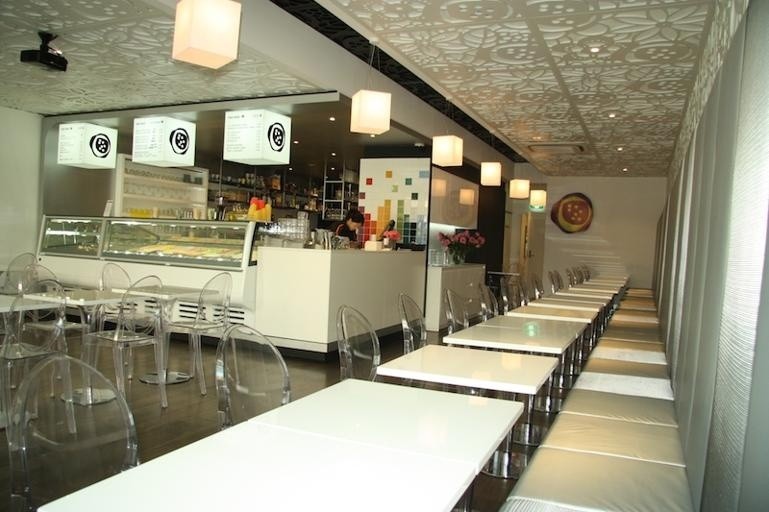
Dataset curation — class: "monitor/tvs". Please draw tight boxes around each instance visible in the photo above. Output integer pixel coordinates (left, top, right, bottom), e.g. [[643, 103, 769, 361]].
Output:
[[377, 220, 395, 241]]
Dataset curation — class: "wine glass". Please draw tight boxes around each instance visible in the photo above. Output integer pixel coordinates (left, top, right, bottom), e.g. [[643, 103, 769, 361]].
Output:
[[327, 207, 341, 219]]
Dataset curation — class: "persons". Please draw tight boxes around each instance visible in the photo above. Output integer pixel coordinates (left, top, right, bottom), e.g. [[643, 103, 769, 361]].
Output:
[[328, 209, 365, 248]]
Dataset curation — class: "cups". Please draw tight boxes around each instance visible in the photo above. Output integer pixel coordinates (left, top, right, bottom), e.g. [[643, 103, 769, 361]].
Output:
[[122, 202, 206, 219], [124, 177, 206, 204], [125, 160, 202, 185]]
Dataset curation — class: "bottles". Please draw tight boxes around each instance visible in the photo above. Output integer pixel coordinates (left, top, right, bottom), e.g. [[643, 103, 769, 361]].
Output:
[[212, 171, 321, 211]]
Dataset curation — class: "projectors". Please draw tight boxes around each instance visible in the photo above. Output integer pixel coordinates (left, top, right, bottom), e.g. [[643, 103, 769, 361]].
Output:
[[20, 48, 68, 71]]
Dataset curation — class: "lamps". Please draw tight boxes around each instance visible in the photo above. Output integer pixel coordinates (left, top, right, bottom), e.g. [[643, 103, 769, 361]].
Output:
[[480, 129, 502, 186], [170, 0, 243, 68], [530, 182, 548, 213], [220, 108, 292, 169], [349, 36, 392, 138], [56, 123, 120, 171], [431, 95, 465, 167], [131, 113, 196, 167]]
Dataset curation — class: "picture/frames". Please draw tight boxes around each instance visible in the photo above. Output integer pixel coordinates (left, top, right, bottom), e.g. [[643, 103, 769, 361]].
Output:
[[550, 192, 593, 233]]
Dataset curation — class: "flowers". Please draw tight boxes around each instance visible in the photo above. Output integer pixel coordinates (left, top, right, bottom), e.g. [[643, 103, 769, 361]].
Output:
[[439, 229, 486, 254]]
